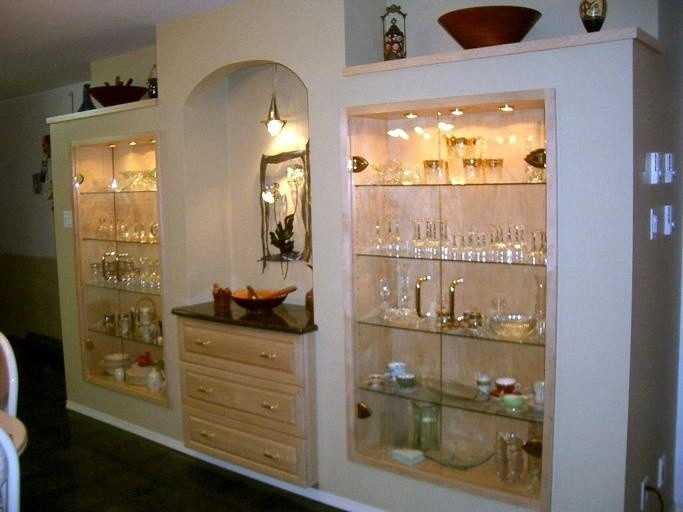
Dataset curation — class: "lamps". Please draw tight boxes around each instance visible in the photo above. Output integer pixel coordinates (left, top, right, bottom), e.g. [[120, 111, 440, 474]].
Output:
[[259, 64, 286, 139]]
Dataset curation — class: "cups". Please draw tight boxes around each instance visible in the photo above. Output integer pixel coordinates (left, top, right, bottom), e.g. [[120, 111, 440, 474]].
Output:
[[95, 219, 160, 244], [424, 158, 502, 183], [388, 362, 416, 394], [476, 373, 543, 413]]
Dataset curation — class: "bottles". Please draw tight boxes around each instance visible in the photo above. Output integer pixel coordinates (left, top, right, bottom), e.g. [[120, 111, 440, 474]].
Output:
[[148, 368, 161, 394], [118, 252, 132, 280], [412, 399, 440, 449], [92, 252, 119, 281]]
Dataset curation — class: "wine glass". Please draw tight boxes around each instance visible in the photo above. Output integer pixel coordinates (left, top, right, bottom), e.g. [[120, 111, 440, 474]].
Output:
[[370, 215, 546, 263], [138, 255, 160, 289]]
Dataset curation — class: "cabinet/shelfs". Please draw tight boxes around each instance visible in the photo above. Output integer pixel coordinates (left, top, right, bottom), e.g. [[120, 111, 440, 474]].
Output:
[[345, 87, 553, 511], [178, 317, 304, 488], [71, 132, 169, 408]]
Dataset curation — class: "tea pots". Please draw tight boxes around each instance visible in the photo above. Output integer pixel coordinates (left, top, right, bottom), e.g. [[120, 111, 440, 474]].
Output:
[[444, 134, 484, 183], [131, 294, 162, 344], [495, 430, 527, 487]]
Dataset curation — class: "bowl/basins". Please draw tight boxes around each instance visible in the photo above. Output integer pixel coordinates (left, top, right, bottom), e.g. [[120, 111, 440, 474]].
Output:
[[492, 312, 539, 339], [424, 440, 496, 471], [232, 290, 288, 309], [88, 86, 149, 106], [103, 354, 131, 376], [438, 5, 542, 52]]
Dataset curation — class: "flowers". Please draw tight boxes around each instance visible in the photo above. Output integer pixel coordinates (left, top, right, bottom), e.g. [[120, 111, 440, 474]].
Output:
[[260, 165, 307, 260]]
